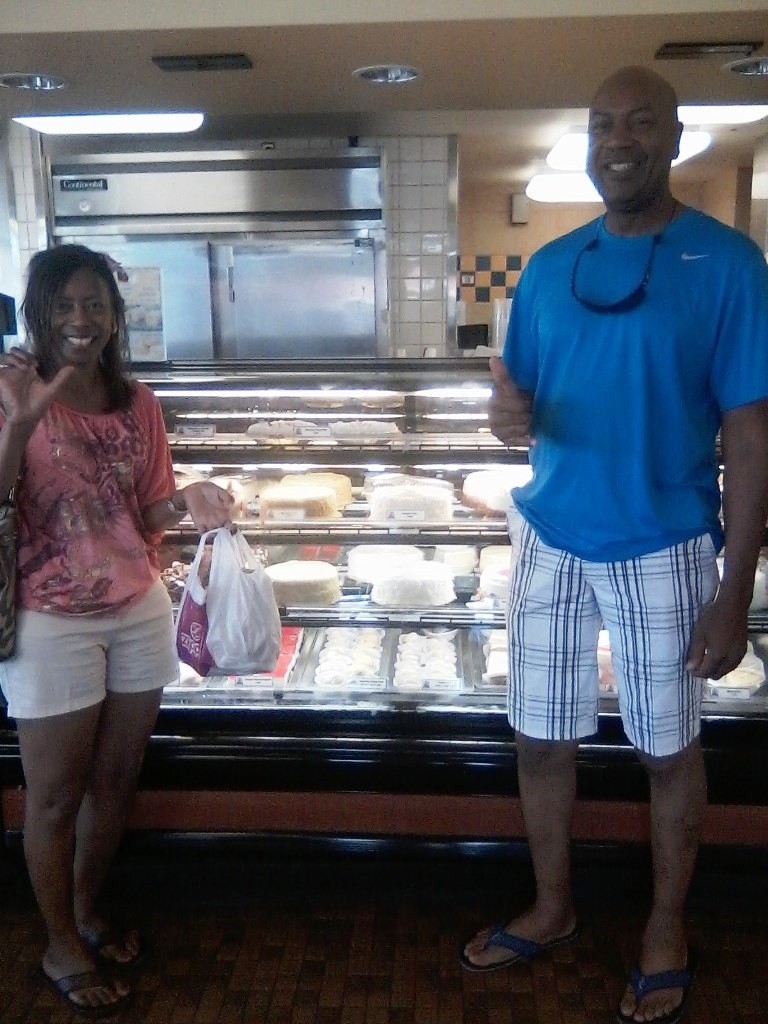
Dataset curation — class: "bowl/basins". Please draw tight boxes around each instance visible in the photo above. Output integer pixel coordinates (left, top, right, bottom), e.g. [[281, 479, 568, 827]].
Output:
[[706, 668, 765, 696], [419, 628, 458, 641]]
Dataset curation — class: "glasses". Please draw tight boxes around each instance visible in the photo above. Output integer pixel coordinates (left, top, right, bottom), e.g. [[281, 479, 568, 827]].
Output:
[[570, 232, 662, 311]]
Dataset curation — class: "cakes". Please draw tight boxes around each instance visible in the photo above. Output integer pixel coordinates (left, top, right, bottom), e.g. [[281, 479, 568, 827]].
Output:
[[265, 544, 513, 609], [257, 469, 534, 522]]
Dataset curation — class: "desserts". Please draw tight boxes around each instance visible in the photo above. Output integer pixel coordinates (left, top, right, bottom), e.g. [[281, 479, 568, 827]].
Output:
[[314, 627, 767, 698]]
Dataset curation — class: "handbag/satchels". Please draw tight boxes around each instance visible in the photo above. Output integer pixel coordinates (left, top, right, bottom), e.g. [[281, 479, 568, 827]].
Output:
[[176, 524, 281, 679], [0, 498, 18, 659]]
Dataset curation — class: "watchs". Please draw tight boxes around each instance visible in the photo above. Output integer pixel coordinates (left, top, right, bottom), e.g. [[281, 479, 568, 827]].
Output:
[[166, 494, 188, 515]]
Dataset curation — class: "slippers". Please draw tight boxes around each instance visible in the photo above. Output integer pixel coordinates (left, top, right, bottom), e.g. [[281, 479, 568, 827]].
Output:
[[77, 923, 141, 968], [456, 915, 579, 973], [616, 933, 694, 1024], [36, 951, 134, 1018]]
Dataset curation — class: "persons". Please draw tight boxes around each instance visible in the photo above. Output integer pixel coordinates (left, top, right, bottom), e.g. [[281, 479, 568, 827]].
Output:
[[455, 65, 768, 1023], [0, 243, 239, 1012]]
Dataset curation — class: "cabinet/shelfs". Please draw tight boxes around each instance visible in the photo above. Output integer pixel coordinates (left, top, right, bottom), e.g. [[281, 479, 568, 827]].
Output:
[[123, 355, 768, 730]]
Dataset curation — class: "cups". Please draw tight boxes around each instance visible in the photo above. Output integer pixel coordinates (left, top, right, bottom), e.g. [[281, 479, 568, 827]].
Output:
[[491, 298, 512, 350]]
